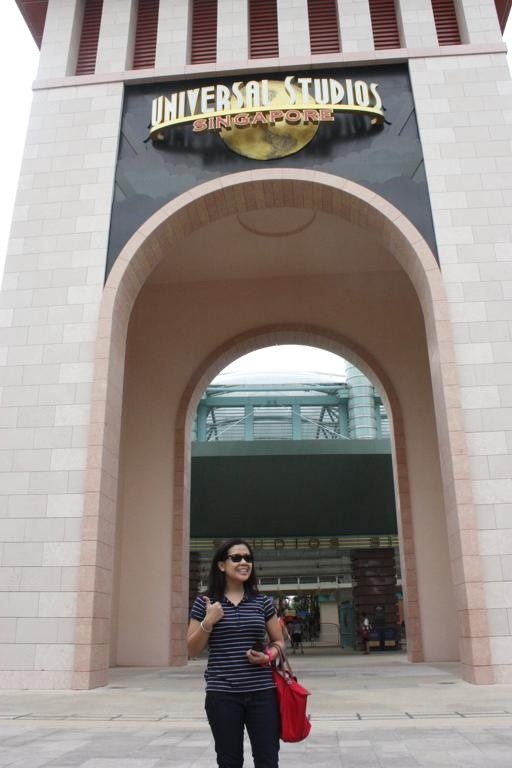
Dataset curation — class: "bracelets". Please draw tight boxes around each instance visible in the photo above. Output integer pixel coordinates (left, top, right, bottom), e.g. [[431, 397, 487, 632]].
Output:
[[199, 620, 214, 632], [259, 645, 274, 669]]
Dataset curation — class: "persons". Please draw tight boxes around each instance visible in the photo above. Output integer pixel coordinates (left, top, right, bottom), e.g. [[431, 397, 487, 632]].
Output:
[[276, 610, 309, 655], [185, 538, 288, 768], [360, 610, 373, 654]]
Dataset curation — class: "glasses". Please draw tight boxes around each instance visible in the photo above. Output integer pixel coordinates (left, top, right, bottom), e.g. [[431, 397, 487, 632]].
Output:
[[222, 553, 253, 563]]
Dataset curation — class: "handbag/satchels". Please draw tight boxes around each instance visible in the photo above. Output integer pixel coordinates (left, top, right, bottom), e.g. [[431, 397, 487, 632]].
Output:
[[270, 665, 311, 743]]
